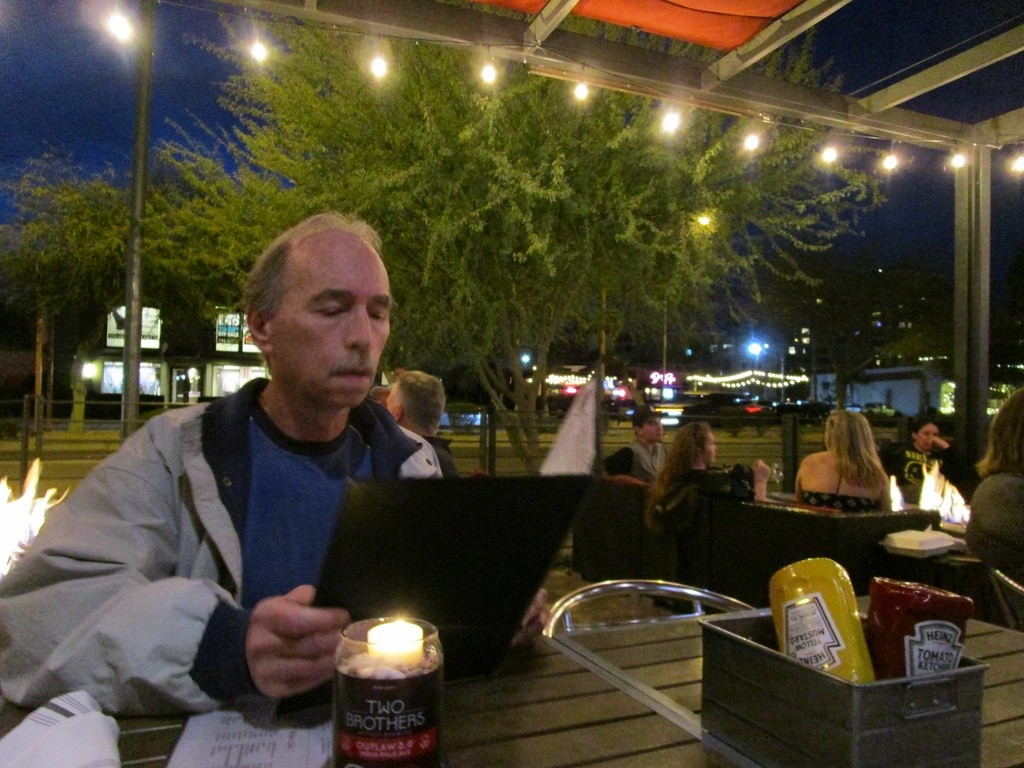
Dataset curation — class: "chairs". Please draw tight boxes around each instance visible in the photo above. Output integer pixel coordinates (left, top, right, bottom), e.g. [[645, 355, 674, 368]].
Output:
[[542, 577, 761, 638], [978, 559, 1024, 632], [575, 475, 652, 583]]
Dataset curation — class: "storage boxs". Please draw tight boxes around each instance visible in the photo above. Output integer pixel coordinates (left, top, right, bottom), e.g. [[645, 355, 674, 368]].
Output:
[[695, 603, 990, 768]]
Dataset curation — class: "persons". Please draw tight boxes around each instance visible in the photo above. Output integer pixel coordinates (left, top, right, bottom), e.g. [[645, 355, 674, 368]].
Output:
[[0, 211, 598, 715], [963, 386, 1024, 631], [882, 408, 966, 493], [793, 407, 893, 515], [600, 409, 774, 547]]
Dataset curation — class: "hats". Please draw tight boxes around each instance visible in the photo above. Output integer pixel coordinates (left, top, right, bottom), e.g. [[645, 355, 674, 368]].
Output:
[[632, 405, 669, 423]]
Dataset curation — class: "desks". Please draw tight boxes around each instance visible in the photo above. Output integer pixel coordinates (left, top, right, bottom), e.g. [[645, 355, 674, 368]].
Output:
[[0, 593, 1024, 768], [771, 492, 973, 534]]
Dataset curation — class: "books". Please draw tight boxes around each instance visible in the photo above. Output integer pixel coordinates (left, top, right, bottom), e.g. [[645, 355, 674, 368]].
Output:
[[308, 355, 595, 673]]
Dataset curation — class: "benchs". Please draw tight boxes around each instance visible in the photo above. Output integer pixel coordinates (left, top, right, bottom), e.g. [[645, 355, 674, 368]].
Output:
[[669, 490, 942, 603]]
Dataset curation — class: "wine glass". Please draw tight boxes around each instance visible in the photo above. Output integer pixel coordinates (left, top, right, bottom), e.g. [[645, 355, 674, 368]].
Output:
[[771, 464, 784, 492]]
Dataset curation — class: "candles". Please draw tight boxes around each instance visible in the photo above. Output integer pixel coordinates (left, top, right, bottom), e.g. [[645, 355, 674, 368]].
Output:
[[366, 621, 424, 666]]
